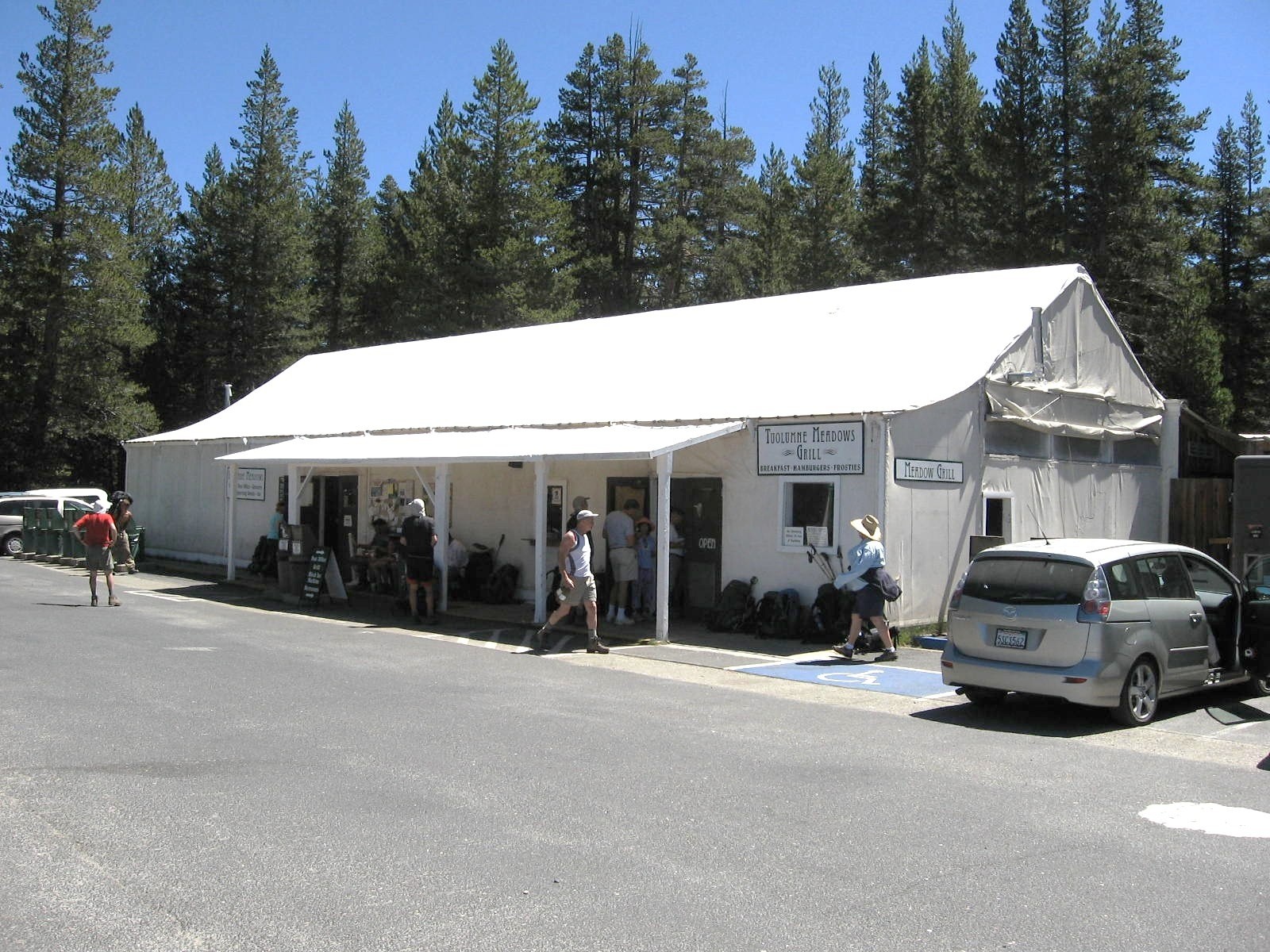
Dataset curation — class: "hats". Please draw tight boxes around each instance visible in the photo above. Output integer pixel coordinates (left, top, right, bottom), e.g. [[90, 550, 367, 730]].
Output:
[[93, 500, 106, 511], [408, 498, 426, 517], [635, 517, 656, 531], [577, 511, 599, 519], [851, 514, 882, 541], [573, 496, 590, 511]]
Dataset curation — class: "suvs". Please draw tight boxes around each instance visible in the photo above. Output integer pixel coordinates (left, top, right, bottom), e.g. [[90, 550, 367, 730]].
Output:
[[0, 487, 108, 556]]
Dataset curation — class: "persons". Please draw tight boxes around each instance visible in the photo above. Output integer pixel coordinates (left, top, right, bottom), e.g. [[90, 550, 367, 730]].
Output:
[[566, 496, 594, 622], [399, 499, 438, 624], [832, 515, 898, 661], [73, 500, 121, 607], [602, 499, 640, 625], [533, 510, 609, 654], [346, 519, 402, 595], [631, 518, 656, 622], [669, 508, 687, 610], [112, 499, 139, 576], [261, 502, 287, 576], [448, 533, 468, 582]]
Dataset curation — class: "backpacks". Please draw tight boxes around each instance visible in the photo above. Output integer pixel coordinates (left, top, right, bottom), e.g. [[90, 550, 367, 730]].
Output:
[[756, 588, 800, 639], [801, 584, 852, 644], [704, 580, 757, 632]]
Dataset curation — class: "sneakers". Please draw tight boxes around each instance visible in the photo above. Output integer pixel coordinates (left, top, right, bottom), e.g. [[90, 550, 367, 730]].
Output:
[[833, 644, 853, 657], [587, 636, 610, 654], [874, 648, 899, 662], [537, 628, 551, 651]]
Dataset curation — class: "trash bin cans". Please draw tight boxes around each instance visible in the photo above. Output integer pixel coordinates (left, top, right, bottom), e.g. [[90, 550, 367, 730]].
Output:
[[22, 507, 146, 561]]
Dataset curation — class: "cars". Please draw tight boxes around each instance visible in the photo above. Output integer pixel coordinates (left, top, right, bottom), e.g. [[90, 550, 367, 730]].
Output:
[[941, 538, 1270, 727]]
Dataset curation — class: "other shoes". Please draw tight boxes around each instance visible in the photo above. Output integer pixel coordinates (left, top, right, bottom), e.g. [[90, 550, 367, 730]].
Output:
[[426, 616, 438, 624], [631, 612, 643, 622], [109, 596, 122, 606], [615, 616, 635, 625], [91, 596, 98, 606], [128, 569, 139, 575], [412, 614, 421, 624], [112, 572, 115, 575], [607, 613, 616, 623], [642, 611, 655, 620]]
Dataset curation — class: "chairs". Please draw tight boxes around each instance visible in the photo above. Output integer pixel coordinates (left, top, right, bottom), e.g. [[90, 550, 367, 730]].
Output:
[[1140, 572, 1160, 590], [1162, 566, 1190, 598]]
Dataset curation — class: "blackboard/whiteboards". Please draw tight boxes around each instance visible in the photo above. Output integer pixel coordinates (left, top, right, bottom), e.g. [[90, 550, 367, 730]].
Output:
[[301, 545, 349, 603]]
